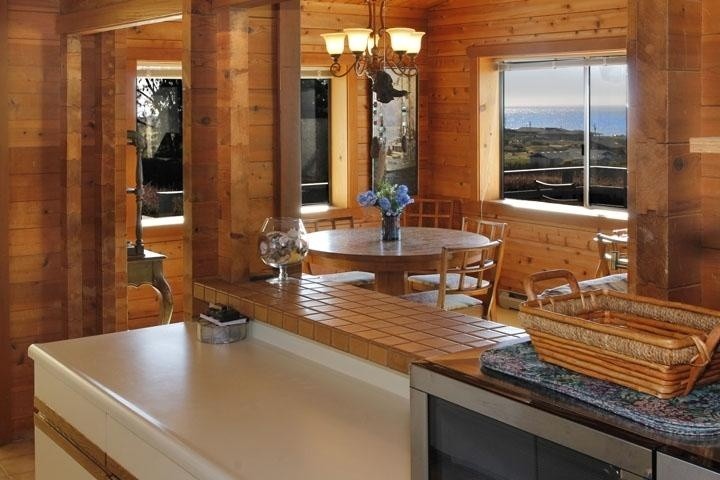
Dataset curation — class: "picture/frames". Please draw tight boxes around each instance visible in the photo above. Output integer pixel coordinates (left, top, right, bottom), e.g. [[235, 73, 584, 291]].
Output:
[[370, 65, 419, 207]]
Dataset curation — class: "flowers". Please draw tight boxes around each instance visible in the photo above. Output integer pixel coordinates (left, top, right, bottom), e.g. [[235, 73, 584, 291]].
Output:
[[358, 184, 415, 216]]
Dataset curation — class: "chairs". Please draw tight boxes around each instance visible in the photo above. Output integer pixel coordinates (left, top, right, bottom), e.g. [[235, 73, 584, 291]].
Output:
[[303, 198, 508, 321], [534, 179, 581, 204]]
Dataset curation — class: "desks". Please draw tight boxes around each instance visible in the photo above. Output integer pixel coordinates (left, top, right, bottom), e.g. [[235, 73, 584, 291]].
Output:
[[126, 249, 174, 325]]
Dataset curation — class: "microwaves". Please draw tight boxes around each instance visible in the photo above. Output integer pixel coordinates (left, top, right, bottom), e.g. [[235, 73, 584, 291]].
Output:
[[407, 362, 719, 480]]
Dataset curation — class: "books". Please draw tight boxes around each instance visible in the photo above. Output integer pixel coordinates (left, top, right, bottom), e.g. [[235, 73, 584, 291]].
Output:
[[199, 311, 249, 326]]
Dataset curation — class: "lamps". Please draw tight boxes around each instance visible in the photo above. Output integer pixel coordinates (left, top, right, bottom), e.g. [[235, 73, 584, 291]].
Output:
[[320, 0, 426, 77]]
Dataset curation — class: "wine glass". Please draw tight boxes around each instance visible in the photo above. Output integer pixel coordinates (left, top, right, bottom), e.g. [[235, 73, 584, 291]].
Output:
[[258, 215, 310, 283]]
